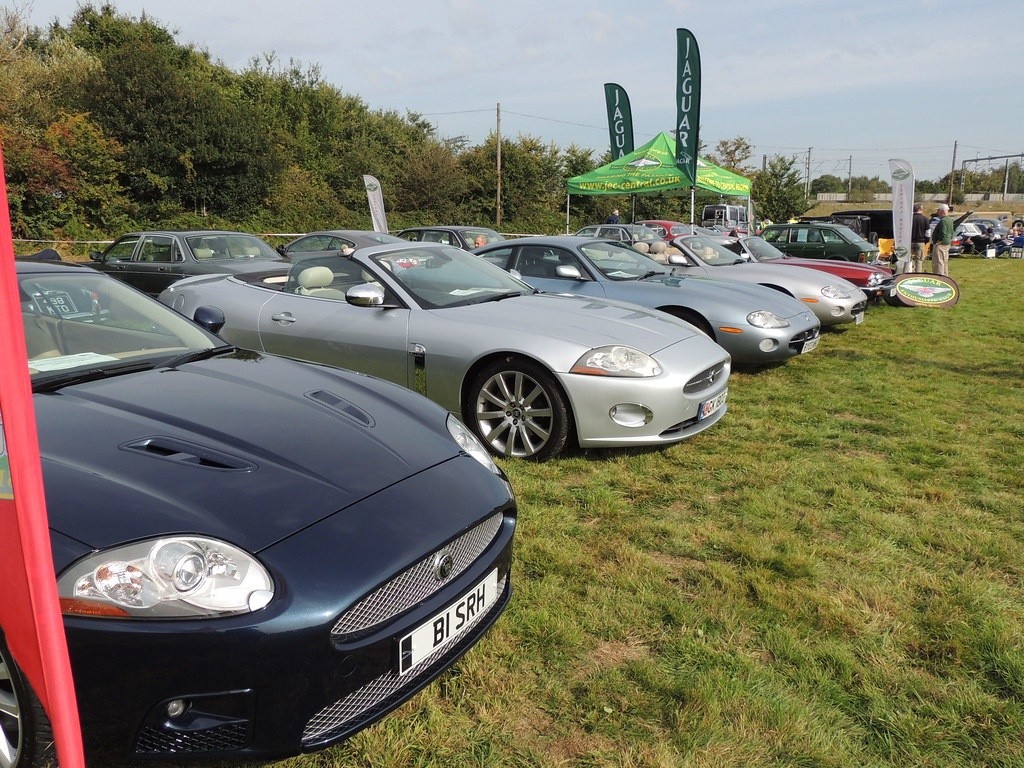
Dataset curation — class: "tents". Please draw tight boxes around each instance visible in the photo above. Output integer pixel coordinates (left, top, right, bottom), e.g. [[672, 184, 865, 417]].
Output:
[[565, 133, 752, 237]]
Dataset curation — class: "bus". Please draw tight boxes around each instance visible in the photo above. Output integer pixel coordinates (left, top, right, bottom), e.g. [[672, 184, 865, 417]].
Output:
[[701, 204, 748, 227]]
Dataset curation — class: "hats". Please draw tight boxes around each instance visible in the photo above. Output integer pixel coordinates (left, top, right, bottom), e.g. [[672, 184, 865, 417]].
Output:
[[934, 204, 949, 210], [986, 227, 994, 231]]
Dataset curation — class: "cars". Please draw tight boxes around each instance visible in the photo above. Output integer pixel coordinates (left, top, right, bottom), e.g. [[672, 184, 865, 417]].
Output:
[[713, 225, 746, 238], [0, 261, 520, 768], [952, 218, 1009, 236], [158, 244, 732, 462], [596, 235, 868, 324], [1011, 213, 1024, 227], [948, 236, 964, 256], [757, 224, 880, 264], [574, 224, 670, 254], [635, 220, 694, 241], [395, 227, 506, 252], [75, 231, 294, 297], [399, 235, 820, 364], [690, 227, 738, 245], [738, 236, 893, 301], [735, 222, 765, 236], [275, 230, 408, 259]]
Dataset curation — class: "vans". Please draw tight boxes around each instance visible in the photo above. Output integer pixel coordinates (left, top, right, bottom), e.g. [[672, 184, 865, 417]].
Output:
[[948, 212, 1012, 229]]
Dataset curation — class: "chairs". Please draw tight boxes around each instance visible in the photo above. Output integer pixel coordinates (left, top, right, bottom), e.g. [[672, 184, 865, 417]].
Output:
[[642, 234, 654, 240], [193, 248, 213, 260], [634, 243, 683, 263], [523, 250, 549, 277], [1006, 245, 1024, 259], [970, 235, 996, 259], [634, 233, 640, 241], [146, 252, 170, 261], [241, 247, 261, 257], [296, 266, 345, 302], [362, 261, 390, 292], [704, 247, 715, 258], [559, 252, 581, 274]]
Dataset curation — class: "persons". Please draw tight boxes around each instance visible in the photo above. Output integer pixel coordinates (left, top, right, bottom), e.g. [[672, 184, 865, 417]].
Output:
[[474, 235, 487, 249], [760, 214, 774, 240], [932, 204, 953, 277], [980, 226, 1024, 257], [606, 209, 620, 233], [903, 202, 931, 274], [787, 213, 798, 224]]
